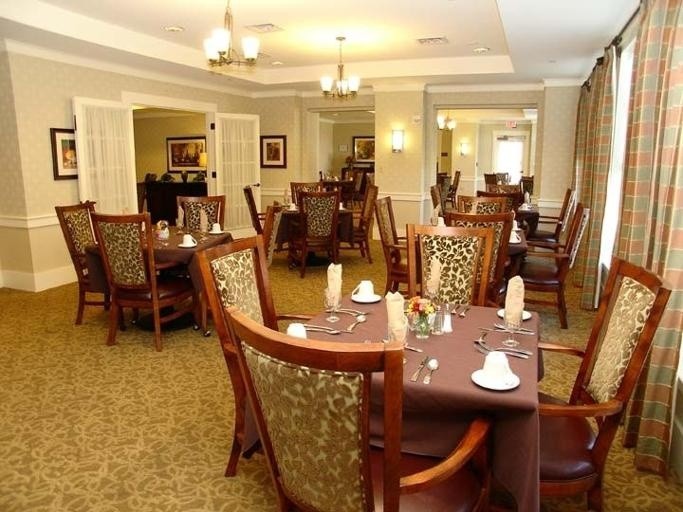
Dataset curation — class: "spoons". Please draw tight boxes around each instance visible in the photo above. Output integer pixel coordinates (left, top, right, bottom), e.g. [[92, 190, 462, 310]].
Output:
[[347, 315, 367, 333], [303, 327, 340, 337], [423, 357, 439, 385]]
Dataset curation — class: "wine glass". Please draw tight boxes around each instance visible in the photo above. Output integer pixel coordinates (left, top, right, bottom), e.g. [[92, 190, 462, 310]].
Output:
[[503, 298, 527, 347], [322, 290, 341, 323], [387, 317, 408, 365]]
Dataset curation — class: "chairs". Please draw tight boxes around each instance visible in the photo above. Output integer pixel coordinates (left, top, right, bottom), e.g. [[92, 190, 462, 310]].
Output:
[[54, 199, 127, 332], [87, 210, 201, 353], [239, 174, 382, 278], [427, 170, 576, 270], [372, 195, 423, 301], [200, 233, 314, 478], [506, 200, 592, 330], [218, 305, 493, 512], [403, 223, 497, 311], [174, 193, 226, 330], [495, 254, 674, 508]]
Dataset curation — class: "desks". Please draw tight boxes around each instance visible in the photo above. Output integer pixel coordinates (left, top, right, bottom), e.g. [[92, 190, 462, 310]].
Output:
[[142, 181, 207, 226], [268, 291, 550, 507]]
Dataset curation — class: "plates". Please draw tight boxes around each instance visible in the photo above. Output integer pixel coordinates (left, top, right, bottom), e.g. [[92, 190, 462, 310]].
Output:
[[351, 295, 382, 304], [472, 369, 522, 391], [338, 208, 345, 211], [497, 308, 532, 323]]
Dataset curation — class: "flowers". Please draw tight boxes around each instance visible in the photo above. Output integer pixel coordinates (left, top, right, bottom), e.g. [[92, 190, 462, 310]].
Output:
[[343, 155, 355, 164]]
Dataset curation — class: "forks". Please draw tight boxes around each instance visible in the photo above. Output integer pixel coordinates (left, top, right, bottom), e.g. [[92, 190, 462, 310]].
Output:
[[479, 340, 533, 355], [408, 356, 429, 382], [325, 308, 375, 316], [450, 302, 461, 314], [301, 323, 353, 334], [493, 321, 532, 333], [471, 342, 528, 361], [458, 304, 473, 319]]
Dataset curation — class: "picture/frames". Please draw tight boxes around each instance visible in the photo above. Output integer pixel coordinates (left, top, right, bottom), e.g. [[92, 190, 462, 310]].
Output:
[[164, 135, 206, 175], [48, 126, 78, 181], [351, 136, 375, 164], [259, 134, 287, 168]]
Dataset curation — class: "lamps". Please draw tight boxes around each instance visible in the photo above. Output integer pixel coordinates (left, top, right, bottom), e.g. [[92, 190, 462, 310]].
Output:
[[203, 0, 262, 75], [391, 130, 403, 154], [318, 36, 362, 103], [436, 110, 458, 130]]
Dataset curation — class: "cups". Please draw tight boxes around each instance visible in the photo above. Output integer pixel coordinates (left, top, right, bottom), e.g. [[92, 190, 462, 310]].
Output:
[[508, 192, 532, 245], [282, 198, 298, 213], [339, 202, 342, 208], [351, 280, 375, 299], [436, 216, 445, 226], [141, 217, 224, 249], [286, 322, 307, 340], [481, 353, 516, 384]]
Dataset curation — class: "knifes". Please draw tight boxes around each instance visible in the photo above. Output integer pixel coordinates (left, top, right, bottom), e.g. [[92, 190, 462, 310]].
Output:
[[381, 338, 425, 354], [475, 325, 534, 338]]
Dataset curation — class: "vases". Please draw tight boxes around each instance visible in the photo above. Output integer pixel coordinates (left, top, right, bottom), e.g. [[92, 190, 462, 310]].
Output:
[[347, 163, 352, 170]]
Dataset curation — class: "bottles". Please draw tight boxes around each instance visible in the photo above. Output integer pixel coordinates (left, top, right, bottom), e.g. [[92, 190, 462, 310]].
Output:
[[431, 305, 444, 335], [442, 301, 454, 333]]
